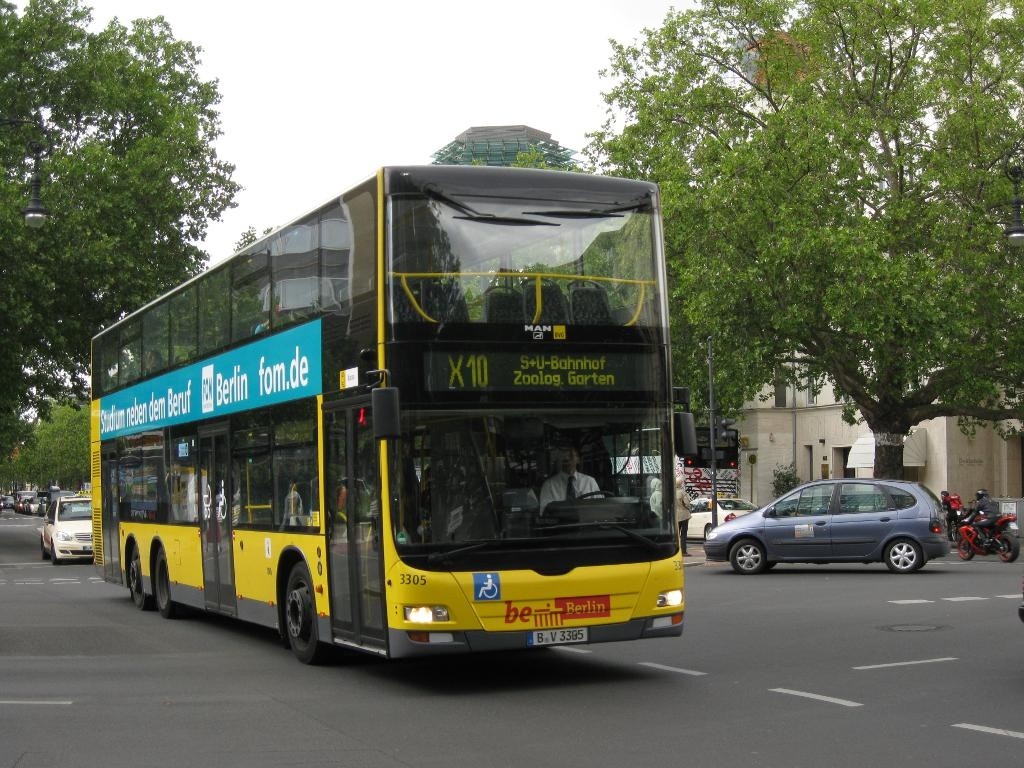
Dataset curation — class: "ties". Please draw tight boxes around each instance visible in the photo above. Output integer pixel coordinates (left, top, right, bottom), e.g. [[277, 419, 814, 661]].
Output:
[[567, 477, 576, 500]]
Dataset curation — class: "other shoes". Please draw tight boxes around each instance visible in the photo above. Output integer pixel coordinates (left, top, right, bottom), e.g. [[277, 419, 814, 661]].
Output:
[[683, 553, 691, 556]]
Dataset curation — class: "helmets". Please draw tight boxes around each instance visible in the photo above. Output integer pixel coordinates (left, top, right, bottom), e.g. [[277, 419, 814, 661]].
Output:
[[976, 489, 986, 500]]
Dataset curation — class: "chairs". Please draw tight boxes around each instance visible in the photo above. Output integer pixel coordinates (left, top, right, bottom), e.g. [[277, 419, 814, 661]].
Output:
[[501, 487, 540, 538], [392, 274, 626, 325]]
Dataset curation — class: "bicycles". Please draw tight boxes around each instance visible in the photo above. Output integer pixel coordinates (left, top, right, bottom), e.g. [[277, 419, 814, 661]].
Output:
[[946, 511, 966, 541]]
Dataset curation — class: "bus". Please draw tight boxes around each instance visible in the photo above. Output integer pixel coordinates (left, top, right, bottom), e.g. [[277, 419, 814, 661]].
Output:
[[90, 165, 688, 667]]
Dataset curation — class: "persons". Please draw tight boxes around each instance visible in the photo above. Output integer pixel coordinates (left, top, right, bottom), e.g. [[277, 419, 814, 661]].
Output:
[[540, 443, 606, 522], [102, 432, 498, 548], [962, 489, 1001, 543], [649, 480, 664, 535], [674, 478, 693, 557], [940, 490, 958, 538]]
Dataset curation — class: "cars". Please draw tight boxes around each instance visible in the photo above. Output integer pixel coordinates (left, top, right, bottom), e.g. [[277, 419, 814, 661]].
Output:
[[40, 497, 93, 563], [0, 489, 76, 516], [703, 478, 949, 574]]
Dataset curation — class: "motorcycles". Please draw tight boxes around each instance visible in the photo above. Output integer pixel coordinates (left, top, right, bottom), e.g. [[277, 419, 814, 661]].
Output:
[[957, 512, 1022, 562]]
